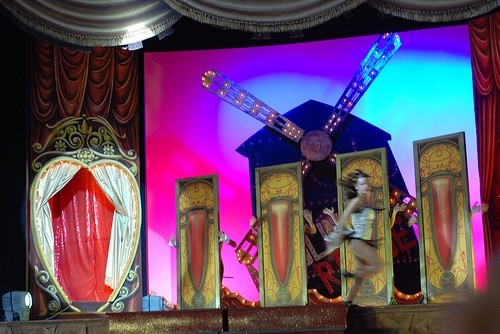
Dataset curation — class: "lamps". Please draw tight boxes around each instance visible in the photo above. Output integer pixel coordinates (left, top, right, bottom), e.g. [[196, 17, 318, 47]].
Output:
[[2, 291, 32, 322]]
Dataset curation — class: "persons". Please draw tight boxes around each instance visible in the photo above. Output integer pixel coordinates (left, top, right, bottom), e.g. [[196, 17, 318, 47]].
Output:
[[407, 201, 488, 228], [335, 170, 382, 307], [167, 229, 237, 248], [323, 204, 408, 228], [249, 210, 317, 234]]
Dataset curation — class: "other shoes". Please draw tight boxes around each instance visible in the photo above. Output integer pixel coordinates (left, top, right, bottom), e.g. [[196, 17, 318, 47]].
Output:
[[343, 272, 354, 278], [342, 300, 353, 307]]
